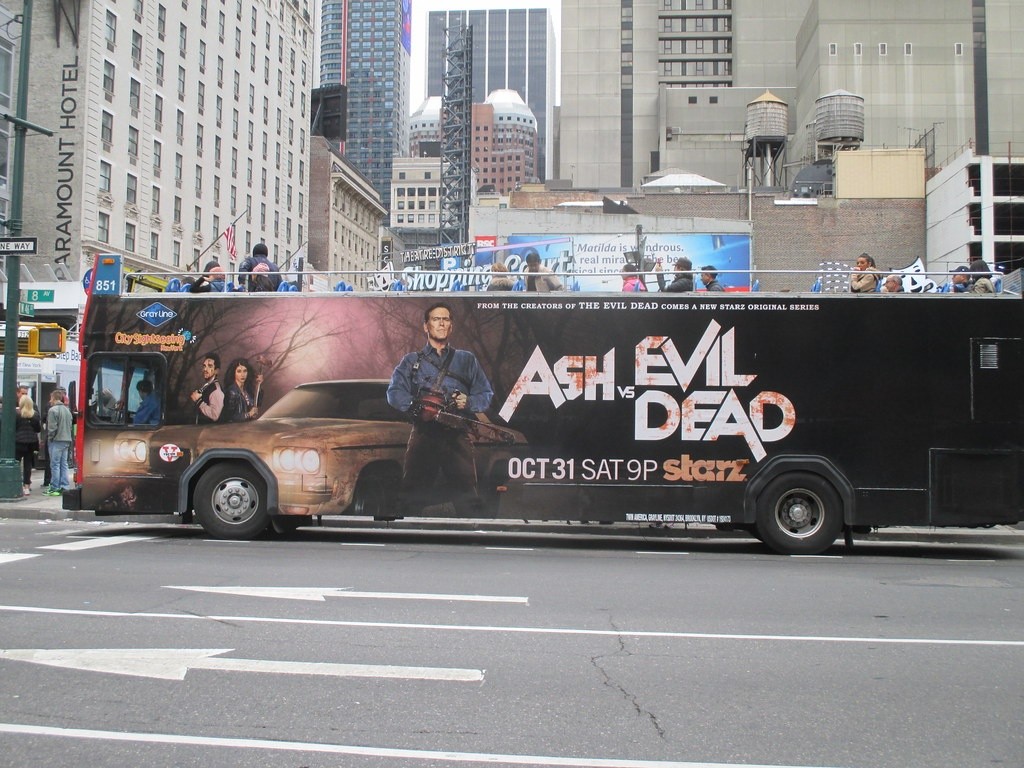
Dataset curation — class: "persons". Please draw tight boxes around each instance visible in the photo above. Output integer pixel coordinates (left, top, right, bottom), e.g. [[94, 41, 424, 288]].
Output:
[[132, 380, 160, 425], [621, 265, 645, 291], [850, 253, 904, 293], [96, 388, 116, 416], [387, 303, 494, 517], [190, 352, 263, 425], [16, 385, 42, 495], [949, 260, 995, 293], [181, 244, 282, 292], [656, 257, 694, 292], [41, 387, 73, 496], [487, 253, 563, 291], [701, 265, 724, 291]]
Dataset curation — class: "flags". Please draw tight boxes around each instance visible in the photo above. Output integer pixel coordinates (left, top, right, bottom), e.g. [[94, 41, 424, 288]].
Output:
[[224, 224, 237, 257], [294, 248, 308, 284]]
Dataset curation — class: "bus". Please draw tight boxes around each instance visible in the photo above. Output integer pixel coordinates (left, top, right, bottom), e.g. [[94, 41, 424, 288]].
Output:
[[62, 252, 1024, 557], [0, 262, 145, 470]]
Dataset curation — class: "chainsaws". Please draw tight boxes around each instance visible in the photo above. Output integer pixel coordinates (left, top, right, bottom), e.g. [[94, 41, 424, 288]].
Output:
[[415, 386, 514, 448]]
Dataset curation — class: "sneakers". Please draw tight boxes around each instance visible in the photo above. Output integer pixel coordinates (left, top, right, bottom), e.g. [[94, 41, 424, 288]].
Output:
[[42, 484, 60, 496]]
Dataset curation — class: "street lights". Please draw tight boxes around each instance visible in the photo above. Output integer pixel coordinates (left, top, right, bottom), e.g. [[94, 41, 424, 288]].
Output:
[[623, 225, 656, 288]]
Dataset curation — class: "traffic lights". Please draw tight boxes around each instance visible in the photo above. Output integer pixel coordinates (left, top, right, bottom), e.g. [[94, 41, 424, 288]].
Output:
[[28, 325, 66, 354]]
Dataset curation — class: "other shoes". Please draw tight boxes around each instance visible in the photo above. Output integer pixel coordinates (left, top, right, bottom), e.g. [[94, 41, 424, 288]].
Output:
[[23, 489, 30, 494]]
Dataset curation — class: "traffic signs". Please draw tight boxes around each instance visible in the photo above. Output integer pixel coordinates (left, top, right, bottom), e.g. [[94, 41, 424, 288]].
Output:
[[0, 237, 38, 255]]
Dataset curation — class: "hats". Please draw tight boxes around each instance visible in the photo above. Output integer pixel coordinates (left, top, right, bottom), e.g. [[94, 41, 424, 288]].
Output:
[[949, 266, 970, 272], [253, 263, 269, 278], [672, 257, 692, 270], [103, 388, 112, 397], [210, 267, 225, 279], [253, 244, 268, 257]]
